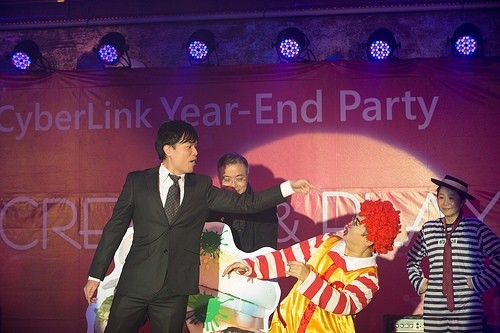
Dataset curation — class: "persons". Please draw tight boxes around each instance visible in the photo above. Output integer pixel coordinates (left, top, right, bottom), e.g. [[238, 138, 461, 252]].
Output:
[[405, 174, 500, 333], [206, 152, 278, 253], [222, 198, 402, 333], [84, 120, 323, 333]]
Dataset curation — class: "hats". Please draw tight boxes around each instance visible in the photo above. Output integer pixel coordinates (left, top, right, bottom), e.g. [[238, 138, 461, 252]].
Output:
[[431, 174, 475, 200]]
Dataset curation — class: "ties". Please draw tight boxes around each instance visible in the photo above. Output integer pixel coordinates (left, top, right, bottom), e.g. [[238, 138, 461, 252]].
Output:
[[163, 173, 182, 221], [440, 212, 462, 311]]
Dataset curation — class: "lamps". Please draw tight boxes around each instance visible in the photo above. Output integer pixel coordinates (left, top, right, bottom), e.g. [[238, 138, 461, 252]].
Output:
[[360, 29, 400, 62], [183, 29, 220, 66], [93, 32, 132, 67], [273, 28, 317, 62], [11, 40, 52, 71], [447, 23, 484, 57]]
